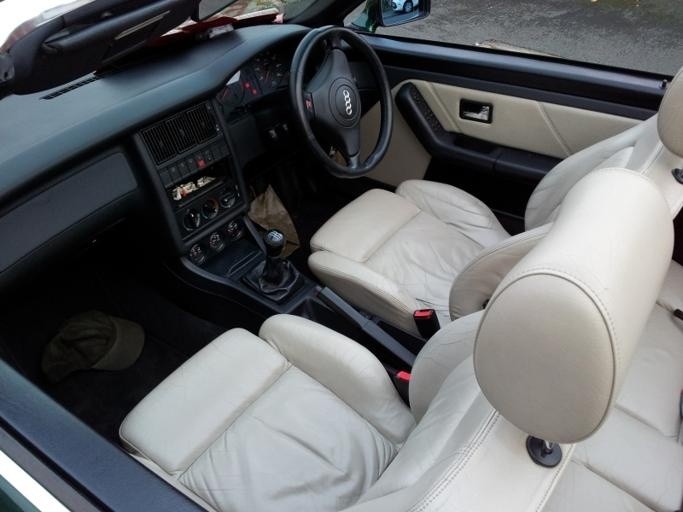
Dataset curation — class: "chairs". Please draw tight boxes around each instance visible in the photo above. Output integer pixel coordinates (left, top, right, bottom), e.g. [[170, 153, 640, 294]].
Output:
[[307, 65, 683, 348], [117, 169, 674, 512]]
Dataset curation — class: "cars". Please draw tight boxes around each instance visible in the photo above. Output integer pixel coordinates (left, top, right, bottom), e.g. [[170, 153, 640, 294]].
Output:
[[391, 1, 419, 14]]
